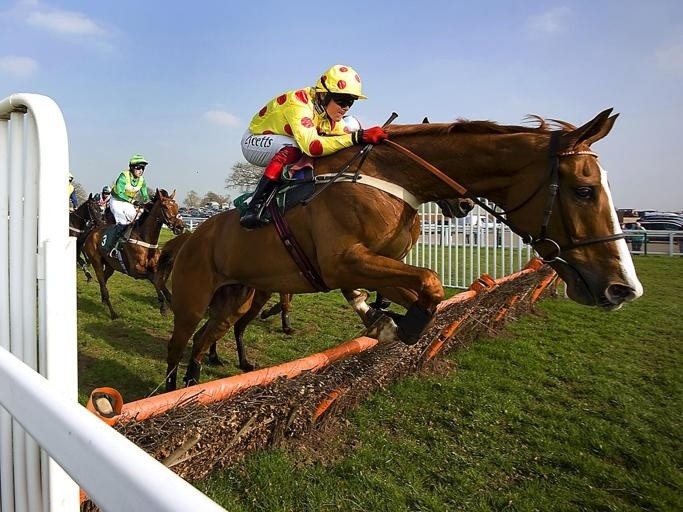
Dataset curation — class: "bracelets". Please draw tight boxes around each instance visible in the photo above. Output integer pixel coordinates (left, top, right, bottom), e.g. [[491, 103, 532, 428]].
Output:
[[351, 130, 358, 146], [129, 198, 133, 203]]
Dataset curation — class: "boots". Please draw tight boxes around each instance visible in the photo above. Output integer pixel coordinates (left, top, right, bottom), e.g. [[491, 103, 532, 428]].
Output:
[[240, 175, 281, 226], [102, 224, 129, 258]]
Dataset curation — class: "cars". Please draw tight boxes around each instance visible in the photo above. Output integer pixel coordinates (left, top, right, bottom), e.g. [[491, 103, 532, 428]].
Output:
[[622, 209, 682, 242], [177, 201, 231, 229]]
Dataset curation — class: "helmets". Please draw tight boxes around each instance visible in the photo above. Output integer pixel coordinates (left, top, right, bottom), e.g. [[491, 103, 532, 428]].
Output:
[[69, 173, 73, 181], [129, 154, 148, 165], [103, 186, 111, 193], [317, 65, 367, 98]]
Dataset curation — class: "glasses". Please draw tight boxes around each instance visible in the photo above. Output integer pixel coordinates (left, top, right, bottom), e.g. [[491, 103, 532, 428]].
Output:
[[321, 81, 354, 108], [129, 165, 144, 171], [105, 193, 111, 195], [69, 180, 72, 183]]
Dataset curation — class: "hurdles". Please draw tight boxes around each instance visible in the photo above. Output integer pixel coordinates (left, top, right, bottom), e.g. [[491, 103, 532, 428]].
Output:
[[79, 257, 562, 512]]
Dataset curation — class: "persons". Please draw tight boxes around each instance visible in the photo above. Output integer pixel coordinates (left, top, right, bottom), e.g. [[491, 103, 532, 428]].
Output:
[[97, 153, 150, 259], [69, 173, 79, 210], [631, 221, 647, 256], [94, 185, 112, 210], [237, 63, 387, 230]]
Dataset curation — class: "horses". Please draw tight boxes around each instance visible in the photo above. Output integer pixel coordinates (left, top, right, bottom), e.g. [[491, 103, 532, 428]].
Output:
[[208, 115, 475, 372], [152, 108, 645, 394], [69, 187, 297, 336]]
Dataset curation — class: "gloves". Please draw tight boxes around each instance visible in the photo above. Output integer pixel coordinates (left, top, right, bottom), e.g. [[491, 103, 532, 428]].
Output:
[[132, 201, 143, 207]]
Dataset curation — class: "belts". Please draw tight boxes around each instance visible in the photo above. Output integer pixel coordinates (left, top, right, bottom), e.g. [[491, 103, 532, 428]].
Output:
[[357, 127, 389, 144]]
